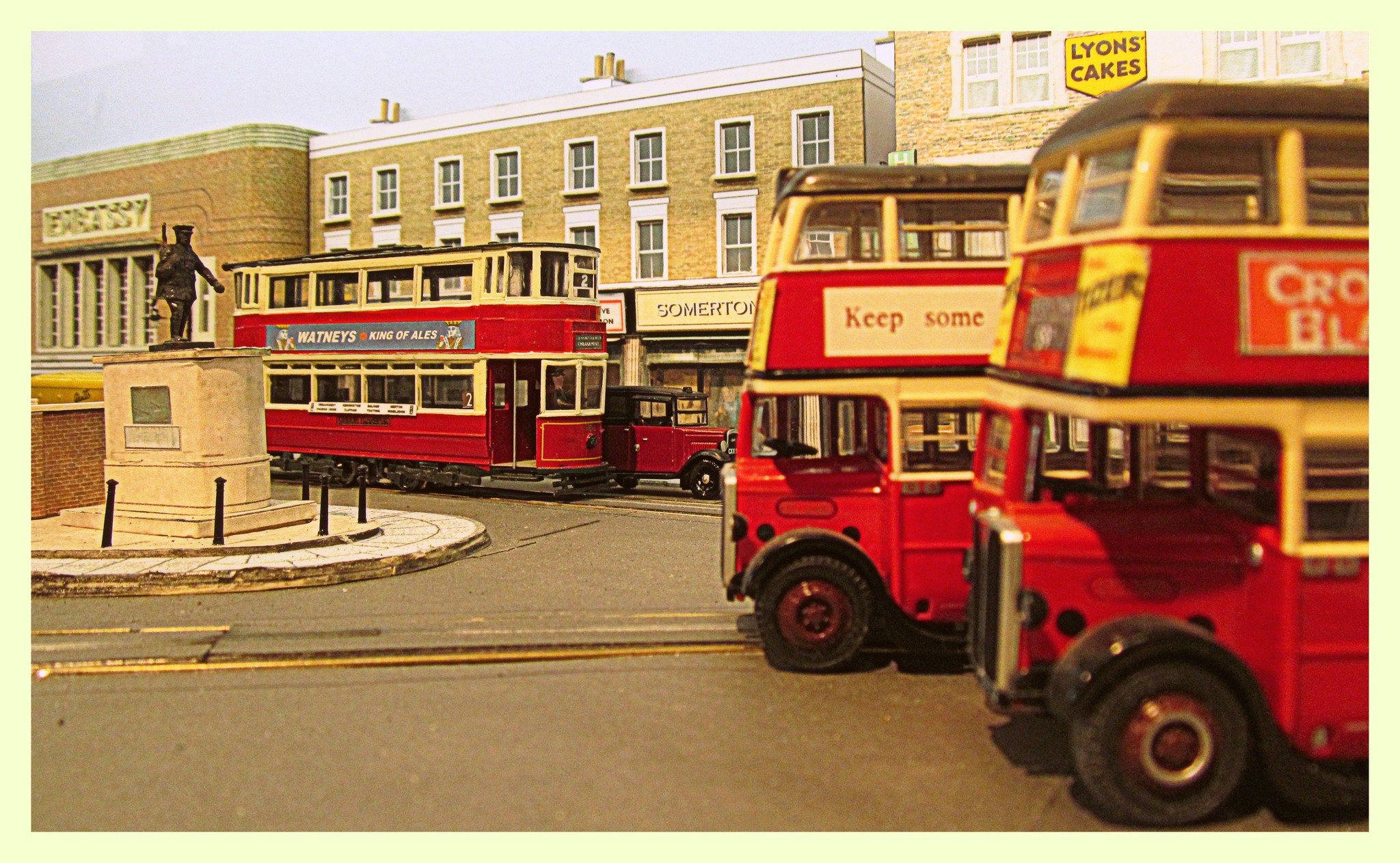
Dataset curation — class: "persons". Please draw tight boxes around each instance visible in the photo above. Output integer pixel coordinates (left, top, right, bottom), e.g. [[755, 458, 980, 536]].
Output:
[[154, 224, 225, 341]]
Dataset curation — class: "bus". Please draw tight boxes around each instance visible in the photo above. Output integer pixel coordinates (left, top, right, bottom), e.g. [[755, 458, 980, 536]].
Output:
[[951, 80, 1369, 824], [221, 240, 614, 500], [720, 149, 1118, 671]]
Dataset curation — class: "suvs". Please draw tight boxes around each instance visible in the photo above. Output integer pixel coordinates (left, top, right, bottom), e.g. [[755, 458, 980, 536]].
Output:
[[583, 382, 740, 499]]
